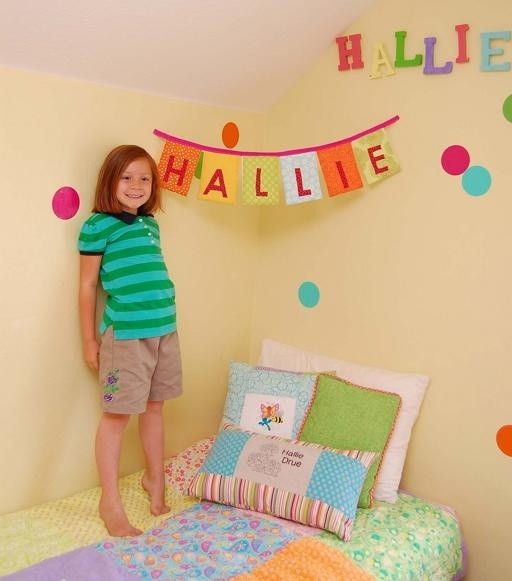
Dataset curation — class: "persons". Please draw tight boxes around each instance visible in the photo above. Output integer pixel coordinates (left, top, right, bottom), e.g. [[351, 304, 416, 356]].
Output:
[[79, 145, 182, 537]]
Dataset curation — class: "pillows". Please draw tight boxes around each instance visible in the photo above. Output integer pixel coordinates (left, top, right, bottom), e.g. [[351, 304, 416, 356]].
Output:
[[257, 338, 432, 506], [295, 372, 402, 511], [183, 420, 378, 544], [221, 358, 338, 446]]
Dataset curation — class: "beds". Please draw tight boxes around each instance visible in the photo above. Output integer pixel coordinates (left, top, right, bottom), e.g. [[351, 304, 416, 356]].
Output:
[[1, 435, 470, 581]]
